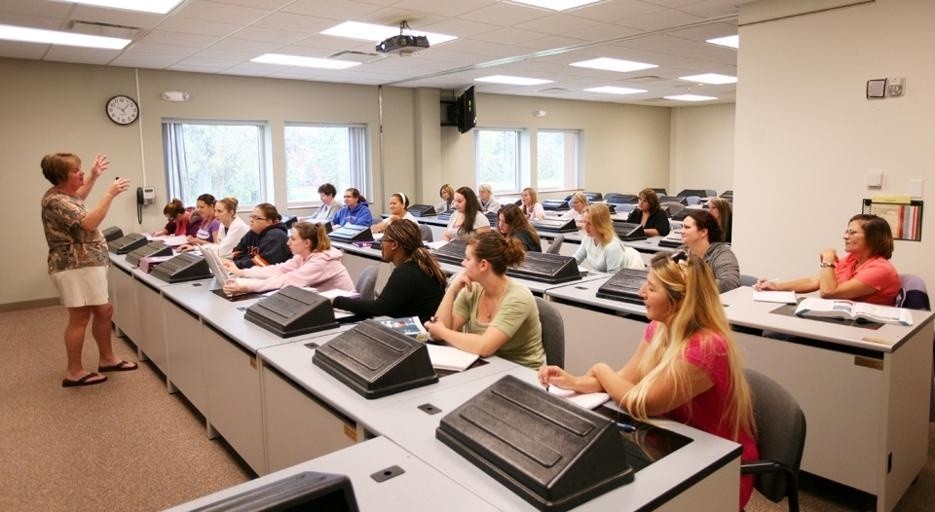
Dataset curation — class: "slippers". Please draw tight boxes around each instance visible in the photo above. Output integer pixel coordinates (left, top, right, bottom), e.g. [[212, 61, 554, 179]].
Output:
[[64, 373, 107, 386], [98, 360, 138, 371]]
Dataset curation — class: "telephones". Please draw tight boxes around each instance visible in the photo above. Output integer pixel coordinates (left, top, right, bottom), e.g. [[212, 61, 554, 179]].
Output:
[[137, 187, 143, 207]]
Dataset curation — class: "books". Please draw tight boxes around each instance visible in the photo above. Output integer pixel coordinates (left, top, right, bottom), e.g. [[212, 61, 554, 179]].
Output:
[[376, 315, 428, 339], [752, 287, 798, 305], [794, 297, 913, 327], [869, 201, 923, 239]]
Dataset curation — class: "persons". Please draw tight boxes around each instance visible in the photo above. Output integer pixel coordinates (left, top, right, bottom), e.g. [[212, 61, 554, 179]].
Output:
[[38, 152, 139, 387], [208, 221, 357, 299], [754, 214, 902, 306], [423, 229, 549, 374], [301, 182, 545, 253], [329, 218, 449, 343], [537, 249, 762, 511], [564, 189, 731, 274], [669, 209, 741, 294], [152, 194, 295, 272]]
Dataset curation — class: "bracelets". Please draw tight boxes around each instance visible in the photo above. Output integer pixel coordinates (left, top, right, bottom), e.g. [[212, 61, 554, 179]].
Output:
[[104, 193, 114, 202]]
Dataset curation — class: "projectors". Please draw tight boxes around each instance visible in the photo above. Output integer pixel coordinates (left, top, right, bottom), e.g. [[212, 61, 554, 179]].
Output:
[[374, 33, 431, 59]]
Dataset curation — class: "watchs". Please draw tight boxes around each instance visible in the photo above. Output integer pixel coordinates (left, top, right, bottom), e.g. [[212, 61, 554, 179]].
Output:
[[820, 259, 836, 268]]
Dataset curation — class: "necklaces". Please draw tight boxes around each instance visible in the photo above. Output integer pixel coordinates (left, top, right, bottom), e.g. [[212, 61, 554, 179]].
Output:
[[481, 284, 505, 320]]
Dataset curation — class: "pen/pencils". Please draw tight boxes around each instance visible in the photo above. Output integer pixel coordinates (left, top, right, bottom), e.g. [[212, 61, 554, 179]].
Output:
[[618, 423, 636, 433], [543, 353, 548, 392]]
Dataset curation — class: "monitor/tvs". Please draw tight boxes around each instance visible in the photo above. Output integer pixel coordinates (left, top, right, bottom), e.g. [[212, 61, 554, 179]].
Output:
[[457, 83, 477, 133]]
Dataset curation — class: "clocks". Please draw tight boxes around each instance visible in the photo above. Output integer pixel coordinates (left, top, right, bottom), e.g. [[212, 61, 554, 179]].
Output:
[[106, 95, 139, 126]]
